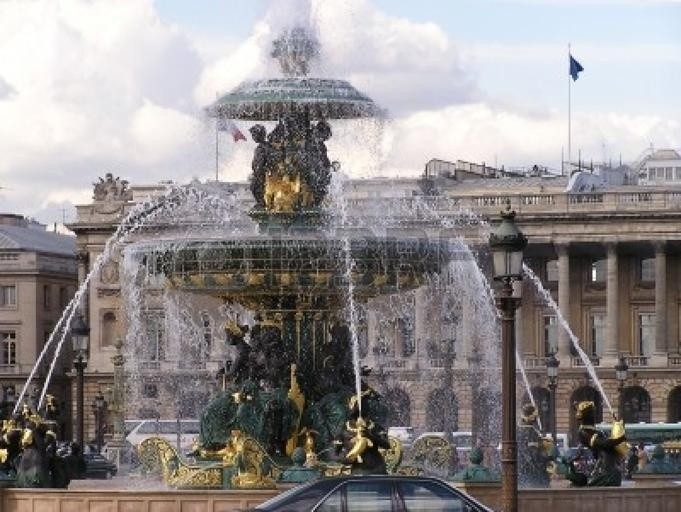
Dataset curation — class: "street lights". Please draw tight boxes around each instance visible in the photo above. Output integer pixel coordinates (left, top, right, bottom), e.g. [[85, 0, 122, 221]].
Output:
[[546, 350, 559, 439], [489, 198, 528, 512], [468, 349, 483, 449], [70, 315, 90, 447], [614, 355, 628, 416]]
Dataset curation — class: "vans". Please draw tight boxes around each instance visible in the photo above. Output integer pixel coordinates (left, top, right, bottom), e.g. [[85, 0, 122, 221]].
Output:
[[124, 419, 200, 452]]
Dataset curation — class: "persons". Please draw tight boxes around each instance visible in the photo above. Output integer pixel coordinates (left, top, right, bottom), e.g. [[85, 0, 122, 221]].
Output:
[[454, 446, 501, 482], [621, 440, 650, 482], [570, 400, 629, 488], [475, 432, 501, 475], [517, 404, 555, 487], [182, 321, 393, 474], [247, 117, 337, 212], [633, 444, 681, 475], [1, 424, 87, 488], [90, 389, 108, 448]]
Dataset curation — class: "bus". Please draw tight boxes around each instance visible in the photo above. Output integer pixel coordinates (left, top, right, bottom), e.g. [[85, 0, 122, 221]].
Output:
[[594, 421, 681, 476]]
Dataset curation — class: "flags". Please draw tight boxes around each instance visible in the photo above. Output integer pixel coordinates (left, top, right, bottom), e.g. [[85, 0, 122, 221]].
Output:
[[216, 118, 248, 143], [569, 53, 586, 83]]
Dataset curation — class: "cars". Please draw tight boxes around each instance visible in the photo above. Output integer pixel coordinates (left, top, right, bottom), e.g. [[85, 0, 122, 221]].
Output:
[[544, 434, 587, 455], [254, 474, 494, 512], [388, 427, 501, 453]]
